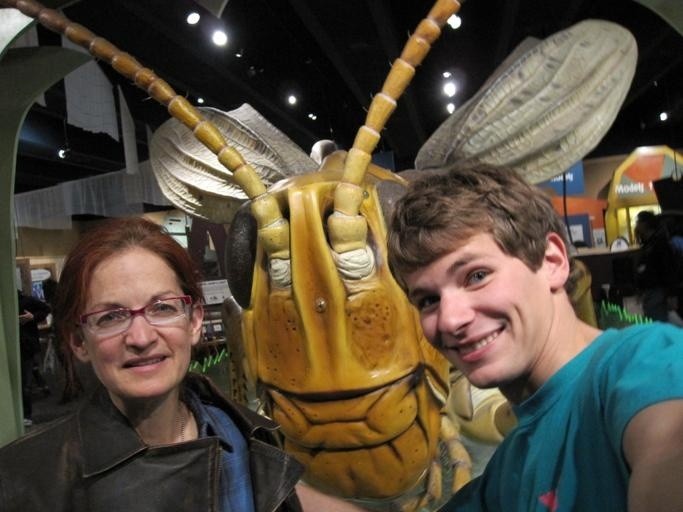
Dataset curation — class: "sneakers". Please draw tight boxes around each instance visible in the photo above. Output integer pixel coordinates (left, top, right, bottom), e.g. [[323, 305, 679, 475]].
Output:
[[23, 417, 34, 429], [58, 386, 80, 406]]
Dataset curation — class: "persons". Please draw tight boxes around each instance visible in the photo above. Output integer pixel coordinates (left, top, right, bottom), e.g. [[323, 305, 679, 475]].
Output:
[[18, 288, 51, 427], [0, 215, 307, 509], [293, 161, 681, 510], [35, 277, 86, 405], [585, 208, 682, 326]]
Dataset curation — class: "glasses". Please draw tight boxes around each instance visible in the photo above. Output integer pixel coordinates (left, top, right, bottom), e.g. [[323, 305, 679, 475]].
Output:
[[72, 295, 198, 340]]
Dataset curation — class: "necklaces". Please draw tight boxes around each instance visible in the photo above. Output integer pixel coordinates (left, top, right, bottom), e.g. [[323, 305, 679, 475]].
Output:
[[179, 414, 185, 443]]
